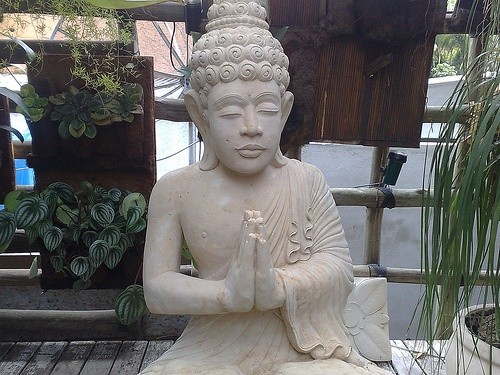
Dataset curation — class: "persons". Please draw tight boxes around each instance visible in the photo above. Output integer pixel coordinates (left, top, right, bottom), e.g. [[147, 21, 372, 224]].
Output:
[[126, 0, 403, 375]]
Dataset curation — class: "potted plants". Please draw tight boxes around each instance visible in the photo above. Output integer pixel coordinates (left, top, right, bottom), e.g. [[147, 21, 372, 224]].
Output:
[[0, 1, 158, 292], [420, 1, 500, 375]]
